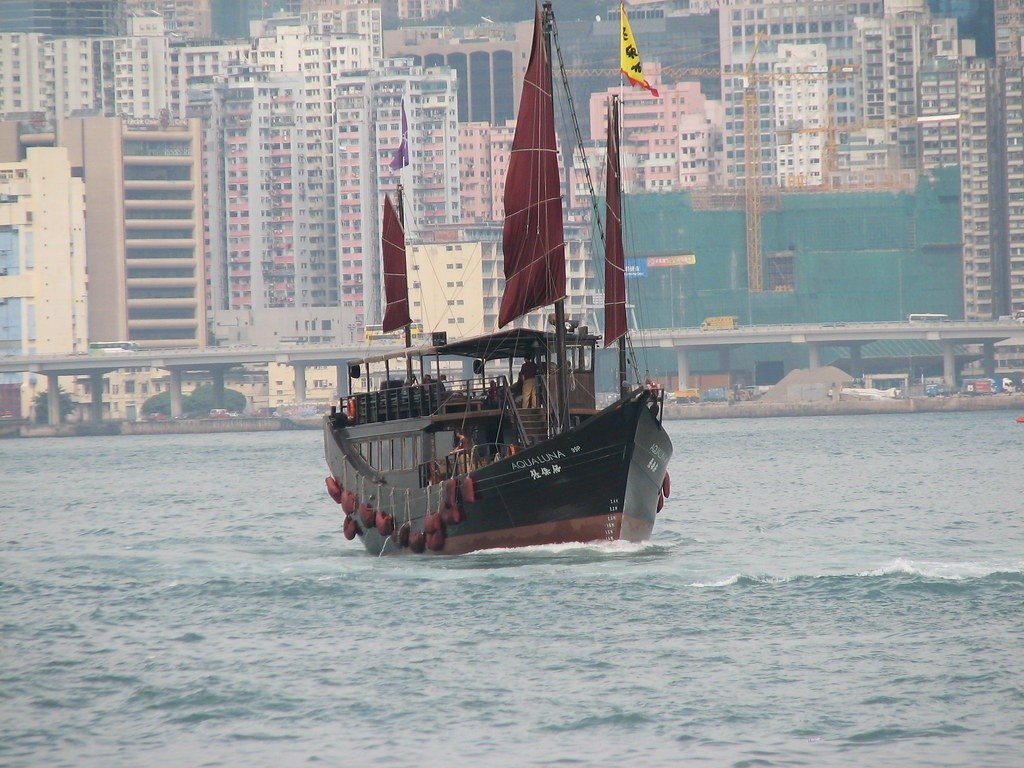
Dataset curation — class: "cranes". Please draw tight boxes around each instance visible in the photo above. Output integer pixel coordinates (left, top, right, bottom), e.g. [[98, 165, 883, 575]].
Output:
[[553, 30, 860, 292], [774, 91, 919, 193]]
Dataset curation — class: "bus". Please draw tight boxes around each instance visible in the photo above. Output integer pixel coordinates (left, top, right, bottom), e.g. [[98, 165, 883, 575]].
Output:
[[908, 313, 949, 325], [88, 341, 139, 357], [363, 322, 423, 345]]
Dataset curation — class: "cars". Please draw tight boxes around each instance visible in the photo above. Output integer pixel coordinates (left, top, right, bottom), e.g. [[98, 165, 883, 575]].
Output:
[[1013, 310, 1024, 323]]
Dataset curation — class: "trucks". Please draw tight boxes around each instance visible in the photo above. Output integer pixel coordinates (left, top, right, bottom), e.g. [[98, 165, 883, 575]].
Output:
[[675, 388, 700, 404], [699, 315, 739, 331]]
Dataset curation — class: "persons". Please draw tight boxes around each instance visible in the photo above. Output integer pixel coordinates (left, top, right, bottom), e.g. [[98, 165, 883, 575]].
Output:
[[450, 429, 470, 474], [402, 353, 571, 409]]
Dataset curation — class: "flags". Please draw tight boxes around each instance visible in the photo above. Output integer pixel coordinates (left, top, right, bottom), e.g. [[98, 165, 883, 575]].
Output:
[[389, 106, 409, 171]]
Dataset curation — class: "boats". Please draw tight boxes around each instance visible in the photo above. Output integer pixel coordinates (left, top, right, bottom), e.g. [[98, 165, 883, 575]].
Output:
[[323, 0, 676, 561]]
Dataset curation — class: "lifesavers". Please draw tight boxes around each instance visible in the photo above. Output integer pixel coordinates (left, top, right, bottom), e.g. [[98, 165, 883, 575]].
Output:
[[506, 444, 515, 457], [347, 399, 354, 420]]
